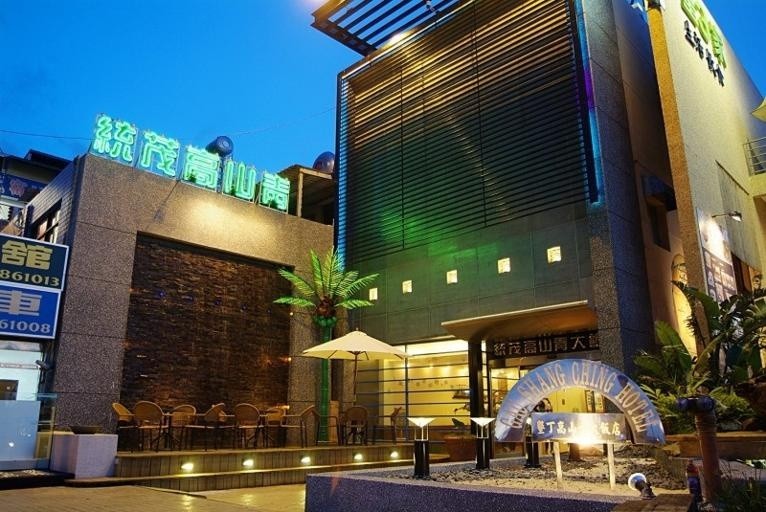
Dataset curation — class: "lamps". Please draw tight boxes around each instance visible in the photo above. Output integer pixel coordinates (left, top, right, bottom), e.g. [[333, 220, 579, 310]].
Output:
[[407, 416, 498, 478], [628, 472, 658, 499], [711, 210, 744, 223]]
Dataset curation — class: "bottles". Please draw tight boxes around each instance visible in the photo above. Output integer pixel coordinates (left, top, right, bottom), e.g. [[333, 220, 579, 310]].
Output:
[[686, 459, 703, 502]]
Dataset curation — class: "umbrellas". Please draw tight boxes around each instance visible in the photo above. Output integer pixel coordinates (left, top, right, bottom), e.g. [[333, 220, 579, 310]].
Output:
[[302, 329, 413, 397]]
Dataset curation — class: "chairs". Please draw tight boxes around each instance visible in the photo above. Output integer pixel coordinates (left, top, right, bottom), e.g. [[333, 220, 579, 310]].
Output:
[[112, 400, 369, 453]]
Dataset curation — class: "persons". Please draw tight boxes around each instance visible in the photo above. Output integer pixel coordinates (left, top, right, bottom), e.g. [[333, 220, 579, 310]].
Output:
[[533, 396, 554, 455]]
